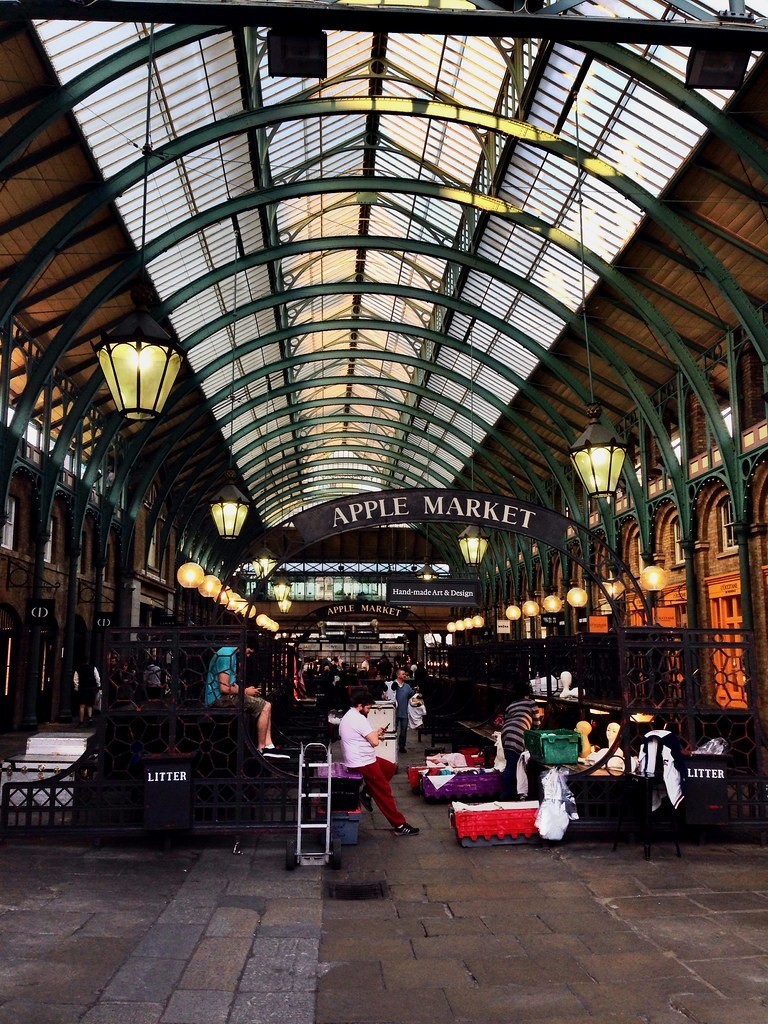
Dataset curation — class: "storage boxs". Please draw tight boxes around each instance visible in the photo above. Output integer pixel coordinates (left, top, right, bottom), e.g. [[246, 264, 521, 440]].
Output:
[[314, 795, 363, 844], [525, 727, 582, 764]]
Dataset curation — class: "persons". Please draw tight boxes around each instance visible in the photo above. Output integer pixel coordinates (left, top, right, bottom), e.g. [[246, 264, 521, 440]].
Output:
[[339, 692, 420, 837], [381, 669, 423, 753], [72, 650, 173, 728], [500, 688, 541, 802], [205, 637, 291, 761], [303, 651, 430, 698], [574, 721, 592, 758], [606, 722, 624, 759]]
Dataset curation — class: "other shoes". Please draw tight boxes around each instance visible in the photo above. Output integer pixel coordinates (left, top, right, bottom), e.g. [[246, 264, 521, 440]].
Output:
[[257, 747, 291, 760], [76, 723, 84, 728], [400, 747, 407, 753]]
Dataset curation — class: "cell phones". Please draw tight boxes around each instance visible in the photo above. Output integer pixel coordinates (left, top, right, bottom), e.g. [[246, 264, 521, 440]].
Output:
[[256, 687, 262, 691], [382, 722, 391, 732]]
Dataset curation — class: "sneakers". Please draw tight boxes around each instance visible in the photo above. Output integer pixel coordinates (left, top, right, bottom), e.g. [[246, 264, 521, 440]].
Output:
[[359, 790, 373, 812], [395, 822, 420, 837]]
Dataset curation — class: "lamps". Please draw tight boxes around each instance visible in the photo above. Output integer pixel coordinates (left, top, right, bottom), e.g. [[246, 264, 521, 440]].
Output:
[[270, 443, 293, 603], [567, 587, 588, 615], [522, 601, 539, 617], [253, 382, 277, 578], [446, 615, 485, 633], [456, 316, 491, 568], [91, 24, 186, 423], [225, 592, 241, 610], [276, 496, 293, 612], [543, 594, 563, 613], [599, 577, 625, 604], [209, 233, 249, 541], [176, 562, 205, 589], [414, 520, 441, 582], [212, 583, 233, 605], [235, 599, 280, 633], [197, 575, 221, 599], [505, 605, 521, 621], [640, 564, 667, 604], [569, 96, 628, 498]]
